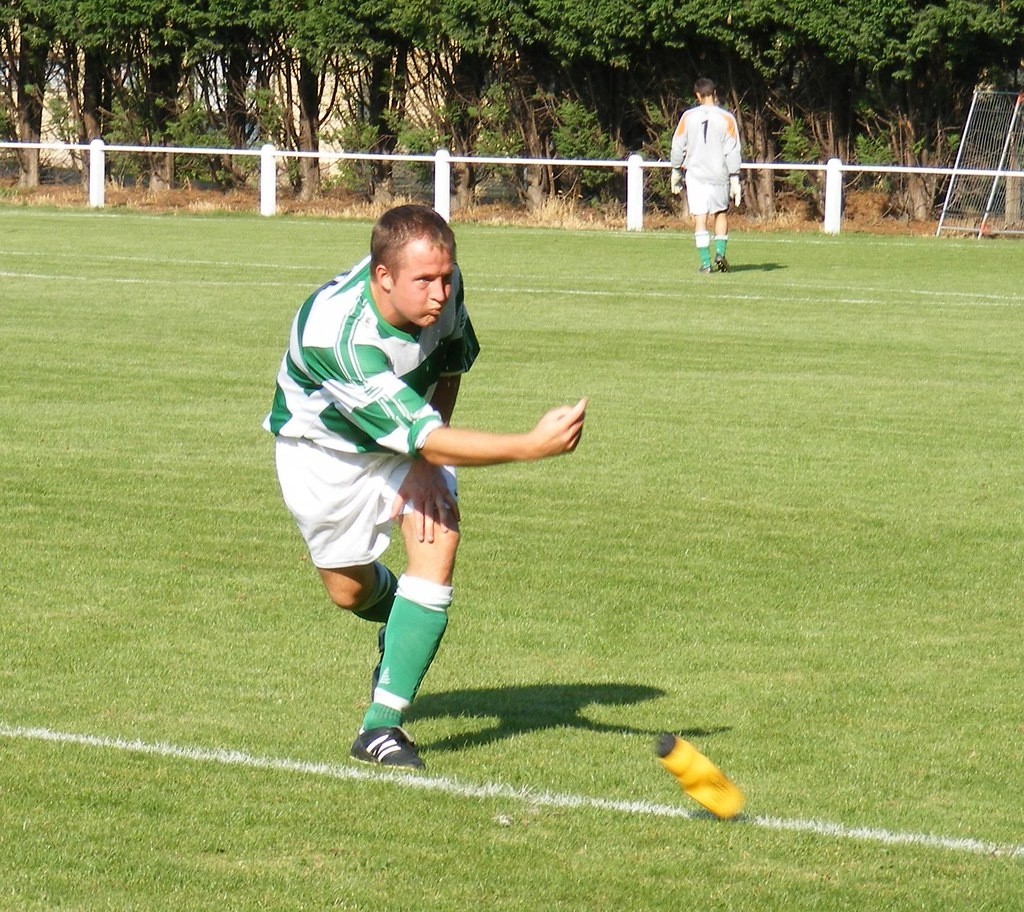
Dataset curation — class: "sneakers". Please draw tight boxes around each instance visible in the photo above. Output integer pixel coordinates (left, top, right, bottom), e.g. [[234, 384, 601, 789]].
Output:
[[371, 626, 387, 702], [350, 726, 425, 772]]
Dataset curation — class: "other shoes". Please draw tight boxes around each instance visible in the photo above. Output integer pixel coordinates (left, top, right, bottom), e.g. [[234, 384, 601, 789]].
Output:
[[715, 254, 729, 273], [699, 266, 714, 273]]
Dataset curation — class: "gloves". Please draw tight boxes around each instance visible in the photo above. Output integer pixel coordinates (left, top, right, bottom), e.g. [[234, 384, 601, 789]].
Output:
[[730, 176, 741, 207], [671, 168, 684, 194]]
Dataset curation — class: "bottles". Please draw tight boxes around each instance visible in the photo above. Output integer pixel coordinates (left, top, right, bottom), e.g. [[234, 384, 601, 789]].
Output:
[[652, 731, 747, 820]]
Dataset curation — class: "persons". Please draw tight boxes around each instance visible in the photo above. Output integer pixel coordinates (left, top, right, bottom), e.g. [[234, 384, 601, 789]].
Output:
[[670, 75, 742, 274], [262, 203, 592, 774]]
